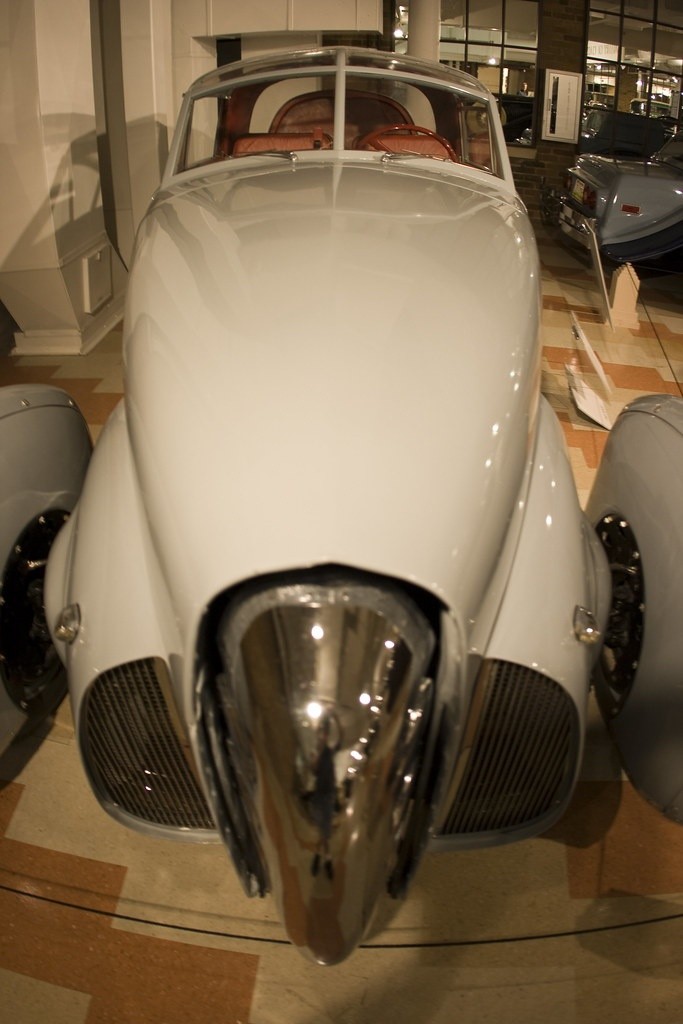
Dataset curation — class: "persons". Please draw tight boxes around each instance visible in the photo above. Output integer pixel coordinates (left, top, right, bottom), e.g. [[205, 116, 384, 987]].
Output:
[[309, 707, 345, 880], [517, 82, 527, 96]]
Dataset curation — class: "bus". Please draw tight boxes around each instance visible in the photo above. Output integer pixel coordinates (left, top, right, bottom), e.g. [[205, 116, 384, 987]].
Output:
[[629, 98, 671, 119]]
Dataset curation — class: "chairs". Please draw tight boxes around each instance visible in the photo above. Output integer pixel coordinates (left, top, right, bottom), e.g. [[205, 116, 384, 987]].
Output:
[[228, 86, 468, 163]]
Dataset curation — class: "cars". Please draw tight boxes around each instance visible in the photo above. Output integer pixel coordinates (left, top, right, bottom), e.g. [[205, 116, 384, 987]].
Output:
[[555, 126, 683, 264]]
